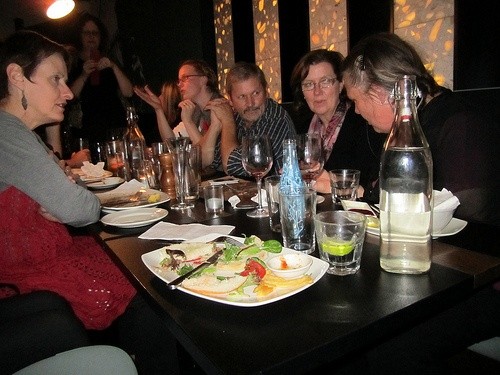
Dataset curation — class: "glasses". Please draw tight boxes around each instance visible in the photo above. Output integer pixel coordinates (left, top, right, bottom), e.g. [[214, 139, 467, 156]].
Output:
[[82, 30, 100, 37], [178, 73, 207, 84], [301, 77, 338, 91]]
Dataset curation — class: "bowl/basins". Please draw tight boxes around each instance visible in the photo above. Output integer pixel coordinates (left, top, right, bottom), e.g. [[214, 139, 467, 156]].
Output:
[[380, 209, 455, 234], [265, 254, 313, 278]]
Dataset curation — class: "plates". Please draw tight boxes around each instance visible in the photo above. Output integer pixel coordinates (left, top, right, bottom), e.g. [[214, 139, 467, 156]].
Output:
[[101, 208, 168, 228], [141, 232, 330, 308], [335, 210, 467, 244], [79, 176, 125, 189], [100, 188, 172, 211]]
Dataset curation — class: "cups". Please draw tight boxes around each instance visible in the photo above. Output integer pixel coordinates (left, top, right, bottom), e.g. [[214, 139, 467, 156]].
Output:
[[313, 211, 367, 276], [264, 175, 282, 232], [203, 185, 225, 214], [278, 187, 317, 254], [76, 135, 201, 210], [329, 169, 361, 205]]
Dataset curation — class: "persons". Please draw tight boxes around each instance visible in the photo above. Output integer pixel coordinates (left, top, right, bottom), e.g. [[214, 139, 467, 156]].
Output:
[[66, 14, 500, 256], [0, 29, 156, 331]]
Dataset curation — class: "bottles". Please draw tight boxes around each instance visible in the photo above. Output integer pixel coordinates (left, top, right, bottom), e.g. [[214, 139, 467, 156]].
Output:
[[279, 139, 306, 239], [123, 107, 145, 182], [378, 74, 432, 275]]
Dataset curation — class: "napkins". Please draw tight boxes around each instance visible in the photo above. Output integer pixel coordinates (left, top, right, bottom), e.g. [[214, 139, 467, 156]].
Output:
[[433, 188, 460, 212], [138, 221, 236, 240], [79, 161, 107, 178]]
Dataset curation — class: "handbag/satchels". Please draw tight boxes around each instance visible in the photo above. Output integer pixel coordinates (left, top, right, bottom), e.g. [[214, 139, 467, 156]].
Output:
[[0, 283, 92, 375]]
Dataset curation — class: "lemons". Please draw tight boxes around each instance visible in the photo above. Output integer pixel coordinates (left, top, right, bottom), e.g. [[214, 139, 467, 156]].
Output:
[[365, 217, 380, 228], [322, 241, 355, 257], [148, 194, 160, 202]]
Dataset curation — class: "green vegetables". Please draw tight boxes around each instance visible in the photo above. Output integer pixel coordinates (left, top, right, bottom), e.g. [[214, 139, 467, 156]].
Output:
[[159, 232, 282, 281]]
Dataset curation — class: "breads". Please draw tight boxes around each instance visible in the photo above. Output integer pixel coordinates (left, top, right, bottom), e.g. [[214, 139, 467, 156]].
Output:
[[184, 266, 248, 293]]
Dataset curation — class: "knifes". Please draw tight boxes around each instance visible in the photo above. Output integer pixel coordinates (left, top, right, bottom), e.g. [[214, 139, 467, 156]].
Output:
[[166, 248, 225, 290]]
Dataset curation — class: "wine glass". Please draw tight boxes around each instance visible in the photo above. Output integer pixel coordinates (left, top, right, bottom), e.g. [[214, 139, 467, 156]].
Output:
[[241, 135, 326, 221]]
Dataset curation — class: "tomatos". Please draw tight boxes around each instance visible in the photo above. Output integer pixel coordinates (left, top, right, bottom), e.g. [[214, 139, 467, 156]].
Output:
[[239, 261, 267, 280]]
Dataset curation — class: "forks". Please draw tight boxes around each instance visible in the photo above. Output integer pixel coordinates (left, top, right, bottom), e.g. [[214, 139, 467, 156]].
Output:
[[151, 236, 245, 248]]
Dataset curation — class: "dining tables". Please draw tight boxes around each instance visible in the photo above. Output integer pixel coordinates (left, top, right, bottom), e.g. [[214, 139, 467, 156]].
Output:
[[61, 156, 500, 375]]
